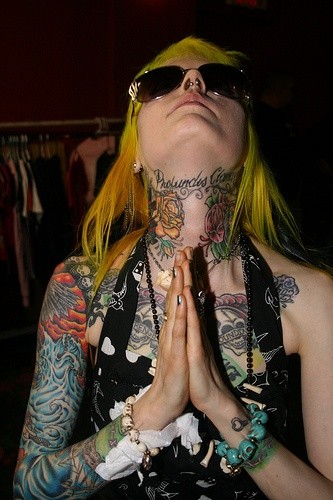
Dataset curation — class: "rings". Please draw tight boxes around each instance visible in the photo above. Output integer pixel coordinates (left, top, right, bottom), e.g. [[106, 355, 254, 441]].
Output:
[[162, 312, 168, 321]]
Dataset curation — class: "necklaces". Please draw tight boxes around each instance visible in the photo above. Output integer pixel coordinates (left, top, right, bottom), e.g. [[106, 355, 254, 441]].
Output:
[[139, 223, 258, 421]]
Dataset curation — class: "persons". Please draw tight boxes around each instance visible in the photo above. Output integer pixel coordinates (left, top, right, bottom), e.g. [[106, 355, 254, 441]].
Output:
[[7, 36, 333, 500]]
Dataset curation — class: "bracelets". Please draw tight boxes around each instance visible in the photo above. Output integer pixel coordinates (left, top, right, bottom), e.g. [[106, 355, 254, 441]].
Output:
[[215, 403, 268, 473], [120, 396, 160, 471]]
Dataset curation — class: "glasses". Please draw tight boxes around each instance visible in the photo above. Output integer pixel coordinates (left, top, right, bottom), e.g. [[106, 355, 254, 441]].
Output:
[[126, 58, 246, 102]]
